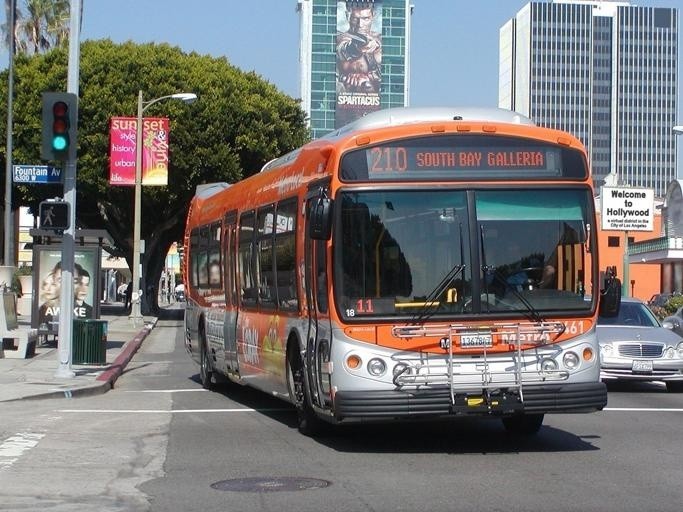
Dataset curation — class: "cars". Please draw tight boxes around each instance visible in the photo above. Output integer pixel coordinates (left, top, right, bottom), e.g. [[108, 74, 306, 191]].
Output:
[[579, 296, 683, 396], [662, 306, 683, 339]]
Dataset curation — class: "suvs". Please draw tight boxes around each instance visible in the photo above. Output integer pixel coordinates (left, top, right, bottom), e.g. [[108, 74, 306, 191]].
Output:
[[644, 292, 682, 311]]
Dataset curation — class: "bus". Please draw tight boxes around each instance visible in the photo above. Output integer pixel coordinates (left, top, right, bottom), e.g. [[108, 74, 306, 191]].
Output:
[[185, 106, 608, 440]]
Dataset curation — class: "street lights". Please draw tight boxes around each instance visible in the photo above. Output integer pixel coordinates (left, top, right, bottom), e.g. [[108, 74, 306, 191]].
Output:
[[670, 124, 682, 135], [126, 85, 200, 324]]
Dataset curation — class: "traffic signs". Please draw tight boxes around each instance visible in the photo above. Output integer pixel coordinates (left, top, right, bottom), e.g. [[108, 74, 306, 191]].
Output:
[[13, 165, 65, 185]]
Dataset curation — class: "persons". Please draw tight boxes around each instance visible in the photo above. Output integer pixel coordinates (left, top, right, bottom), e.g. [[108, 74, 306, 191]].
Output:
[[116, 280, 176, 314], [482, 240, 557, 297], [335, 1, 382, 92], [39, 261, 93, 329]]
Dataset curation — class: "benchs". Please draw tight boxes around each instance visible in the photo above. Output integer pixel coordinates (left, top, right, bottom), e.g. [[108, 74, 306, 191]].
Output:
[[0, 292, 38, 359]]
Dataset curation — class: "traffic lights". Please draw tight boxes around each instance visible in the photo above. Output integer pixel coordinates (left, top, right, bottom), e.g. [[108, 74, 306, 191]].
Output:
[[52, 99, 71, 154], [38, 200, 72, 230]]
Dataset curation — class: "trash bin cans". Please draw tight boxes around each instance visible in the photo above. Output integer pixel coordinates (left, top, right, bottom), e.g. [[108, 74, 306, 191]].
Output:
[[72, 319, 108, 365]]
